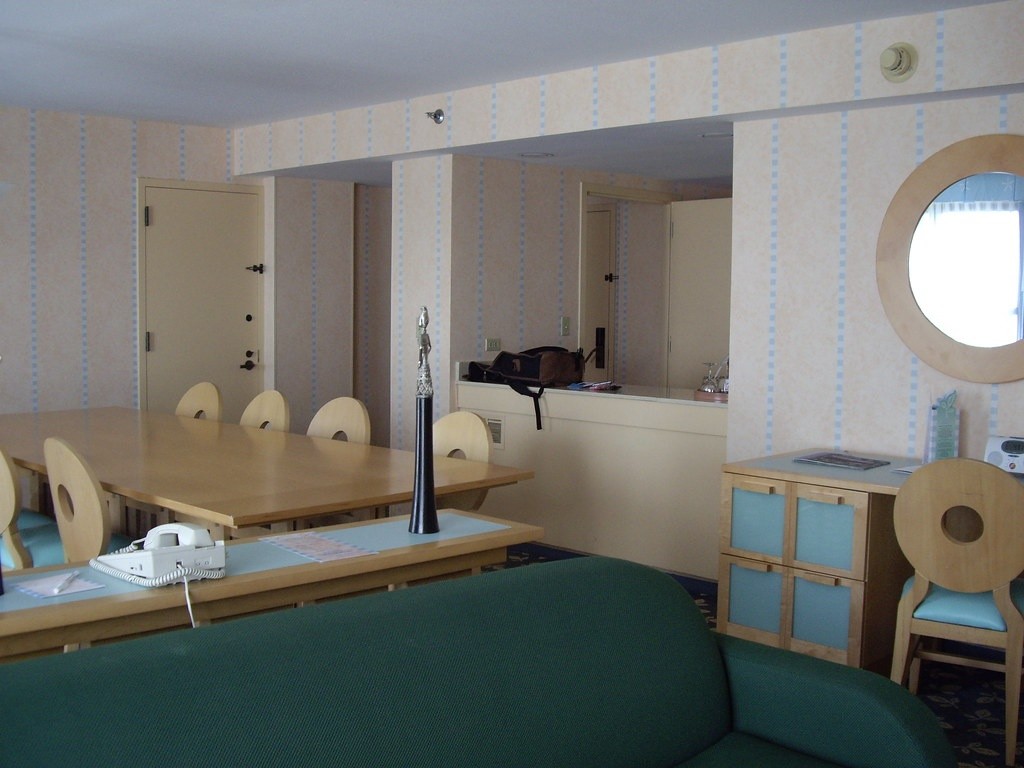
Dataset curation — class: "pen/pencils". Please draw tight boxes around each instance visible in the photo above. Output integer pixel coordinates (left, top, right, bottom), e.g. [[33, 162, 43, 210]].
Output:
[[53, 571, 80, 594]]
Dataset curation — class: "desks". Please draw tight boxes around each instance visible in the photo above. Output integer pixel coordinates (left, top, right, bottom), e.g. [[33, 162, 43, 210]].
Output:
[[0, 406, 532, 542], [0, 508, 541, 663], [715, 451, 1023, 667]]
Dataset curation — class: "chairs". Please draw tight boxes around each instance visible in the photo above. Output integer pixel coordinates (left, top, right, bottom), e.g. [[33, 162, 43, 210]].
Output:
[[44, 436, 111, 560], [306, 399, 371, 446], [889, 458, 1023, 767], [0, 446, 132, 572], [174, 383, 225, 423], [237, 390, 291, 432], [384, 409, 495, 517]]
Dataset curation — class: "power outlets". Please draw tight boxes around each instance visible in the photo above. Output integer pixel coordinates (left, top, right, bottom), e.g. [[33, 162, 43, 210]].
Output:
[[484, 338, 501, 351]]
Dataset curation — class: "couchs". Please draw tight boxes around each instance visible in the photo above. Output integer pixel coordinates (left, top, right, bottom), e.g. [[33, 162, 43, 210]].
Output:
[[0, 555, 954, 768]]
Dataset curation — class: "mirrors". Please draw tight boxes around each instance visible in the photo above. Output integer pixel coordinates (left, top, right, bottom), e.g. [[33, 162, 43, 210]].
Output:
[[876, 133, 1023, 385]]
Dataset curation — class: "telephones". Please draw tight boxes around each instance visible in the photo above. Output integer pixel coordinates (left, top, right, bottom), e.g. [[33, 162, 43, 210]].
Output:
[[88, 522, 227, 588]]
